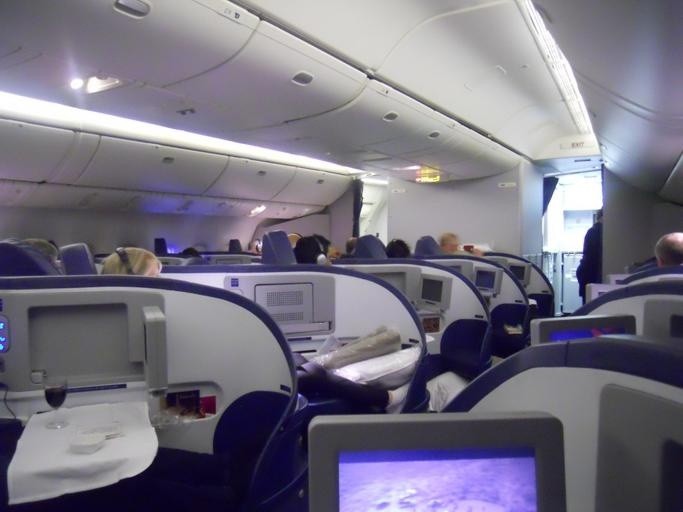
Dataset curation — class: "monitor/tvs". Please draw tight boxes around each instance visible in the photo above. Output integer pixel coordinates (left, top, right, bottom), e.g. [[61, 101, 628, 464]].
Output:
[[585, 283, 621, 302], [531, 314, 636, 344], [509, 262, 531, 285], [421, 274, 452, 307], [308, 410, 568, 512], [476, 267, 502, 292]]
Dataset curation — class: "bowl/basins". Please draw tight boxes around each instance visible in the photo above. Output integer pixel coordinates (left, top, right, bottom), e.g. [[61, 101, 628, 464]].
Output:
[[71, 434, 106, 454]]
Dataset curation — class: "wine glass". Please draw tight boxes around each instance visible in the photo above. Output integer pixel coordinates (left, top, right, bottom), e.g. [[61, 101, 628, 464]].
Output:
[[43, 372, 66, 430]]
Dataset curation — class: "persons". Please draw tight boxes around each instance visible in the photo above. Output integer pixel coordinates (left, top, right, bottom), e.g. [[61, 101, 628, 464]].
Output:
[[385, 238, 409, 258], [247, 237, 263, 254], [294, 234, 331, 264], [98, 244, 163, 277], [574, 206, 604, 306], [285, 232, 303, 249], [343, 237, 358, 254], [652, 230, 682, 268], [20, 238, 59, 266], [439, 232, 461, 254]]
